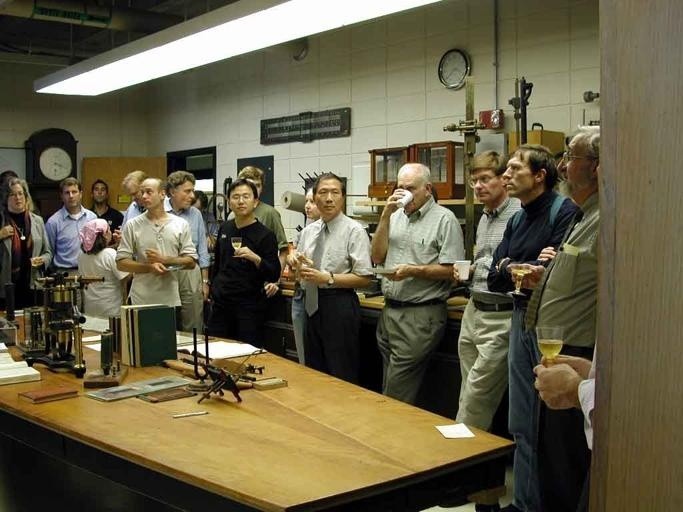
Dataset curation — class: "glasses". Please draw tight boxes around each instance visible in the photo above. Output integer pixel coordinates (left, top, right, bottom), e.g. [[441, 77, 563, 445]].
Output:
[[468, 173, 503, 188], [563, 153, 584, 163]]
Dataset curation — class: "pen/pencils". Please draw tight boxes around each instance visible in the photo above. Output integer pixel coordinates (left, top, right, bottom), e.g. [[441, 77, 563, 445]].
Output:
[[172, 411, 209, 418]]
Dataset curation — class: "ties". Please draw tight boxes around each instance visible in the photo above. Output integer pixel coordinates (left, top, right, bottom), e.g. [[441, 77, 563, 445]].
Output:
[[525, 209, 582, 332], [304, 222, 326, 317]]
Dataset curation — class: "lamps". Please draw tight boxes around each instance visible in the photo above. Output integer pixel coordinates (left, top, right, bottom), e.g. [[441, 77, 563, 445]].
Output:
[[33, 0, 445, 99]]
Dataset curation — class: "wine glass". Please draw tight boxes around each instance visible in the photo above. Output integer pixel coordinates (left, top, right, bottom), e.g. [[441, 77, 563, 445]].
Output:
[[506, 260, 531, 297], [231, 237, 242, 257], [534, 307, 565, 368]]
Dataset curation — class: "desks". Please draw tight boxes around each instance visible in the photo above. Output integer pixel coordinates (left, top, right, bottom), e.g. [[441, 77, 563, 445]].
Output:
[[0, 315, 516, 510], [277, 284, 468, 403]]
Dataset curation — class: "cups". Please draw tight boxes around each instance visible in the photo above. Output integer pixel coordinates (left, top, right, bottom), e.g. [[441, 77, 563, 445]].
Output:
[[456, 260, 471, 281], [395, 191, 413, 208]]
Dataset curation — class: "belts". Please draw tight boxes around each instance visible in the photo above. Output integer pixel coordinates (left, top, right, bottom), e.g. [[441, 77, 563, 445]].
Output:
[[475, 302, 512, 311], [388, 300, 442, 307]]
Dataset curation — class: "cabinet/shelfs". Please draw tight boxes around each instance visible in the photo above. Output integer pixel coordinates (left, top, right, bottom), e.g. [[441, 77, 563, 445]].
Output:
[[367, 146, 410, 200], [411, 141, 464, 199]]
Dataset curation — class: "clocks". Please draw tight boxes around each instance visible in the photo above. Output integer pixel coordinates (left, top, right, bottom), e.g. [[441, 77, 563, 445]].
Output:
[[438, 49, 472, 91], [24, 127, 81, 222]]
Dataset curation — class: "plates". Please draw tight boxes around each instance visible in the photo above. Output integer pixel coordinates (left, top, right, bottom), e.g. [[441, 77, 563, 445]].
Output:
[[368, 267, 396, 275], [164, 264, 186, 271]]
